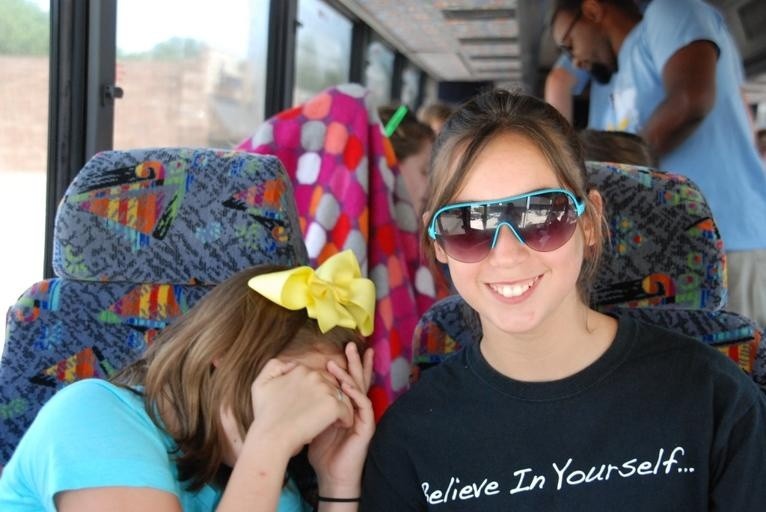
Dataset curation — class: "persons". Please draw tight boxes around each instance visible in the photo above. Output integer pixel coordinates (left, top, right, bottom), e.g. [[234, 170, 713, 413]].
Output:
[[544, 0, 765, 337], [0, 248, 377, 511], [376, 107, 433, 223], [415, 102, 455, 136], [359, 92, 766, 510]]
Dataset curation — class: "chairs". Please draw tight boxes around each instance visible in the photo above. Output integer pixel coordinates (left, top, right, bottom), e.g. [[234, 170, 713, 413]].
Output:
[[408, 159, 764, 406], [0, 146, 311, 471]]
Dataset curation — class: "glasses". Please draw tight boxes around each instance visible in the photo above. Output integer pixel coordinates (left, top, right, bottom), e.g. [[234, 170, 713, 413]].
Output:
[[426, 186, 586, 264]]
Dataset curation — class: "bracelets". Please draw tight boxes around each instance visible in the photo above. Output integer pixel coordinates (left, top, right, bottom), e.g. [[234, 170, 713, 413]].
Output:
[[317, 494, 359, 506]]
